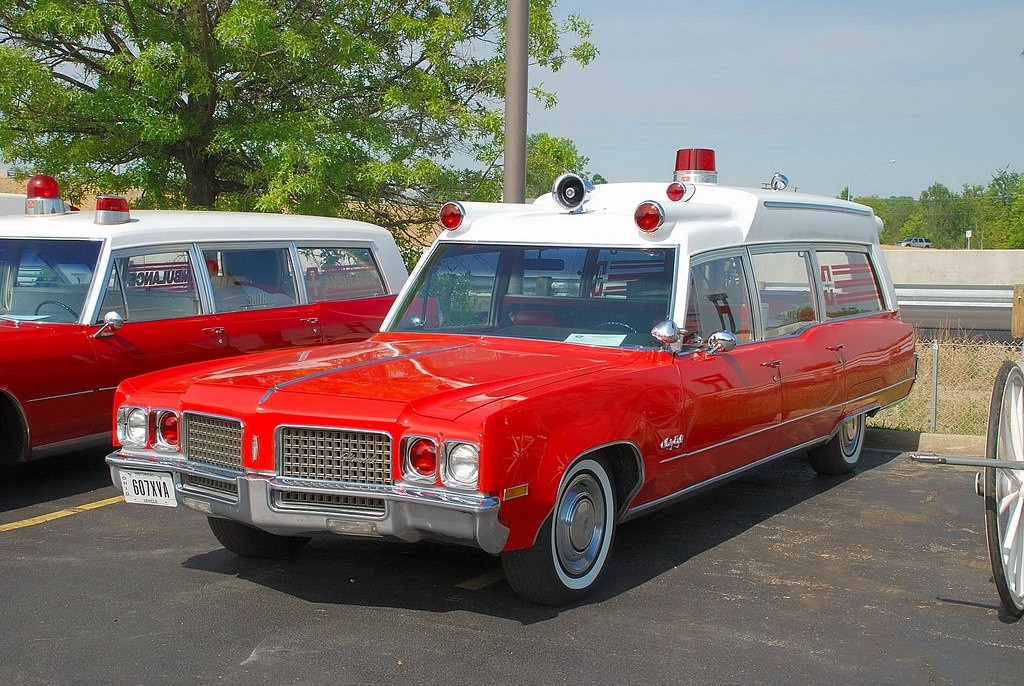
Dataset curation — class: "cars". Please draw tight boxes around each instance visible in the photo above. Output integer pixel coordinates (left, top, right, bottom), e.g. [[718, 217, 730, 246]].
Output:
[[0, 170, 439, 492], [102, 142, 918, 590]]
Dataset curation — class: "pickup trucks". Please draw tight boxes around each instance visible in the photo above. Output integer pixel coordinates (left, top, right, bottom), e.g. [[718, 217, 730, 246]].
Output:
[[894, 238, 932, 249]]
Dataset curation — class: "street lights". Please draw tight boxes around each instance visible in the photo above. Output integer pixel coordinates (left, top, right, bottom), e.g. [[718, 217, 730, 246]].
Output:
[[848, 159, 897, 203]]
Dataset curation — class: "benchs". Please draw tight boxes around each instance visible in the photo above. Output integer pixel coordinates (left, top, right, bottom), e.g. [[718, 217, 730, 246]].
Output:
[[211, 285, 298, 312], [498, 293, 749, 343], [10, 286, 195, 323]]
